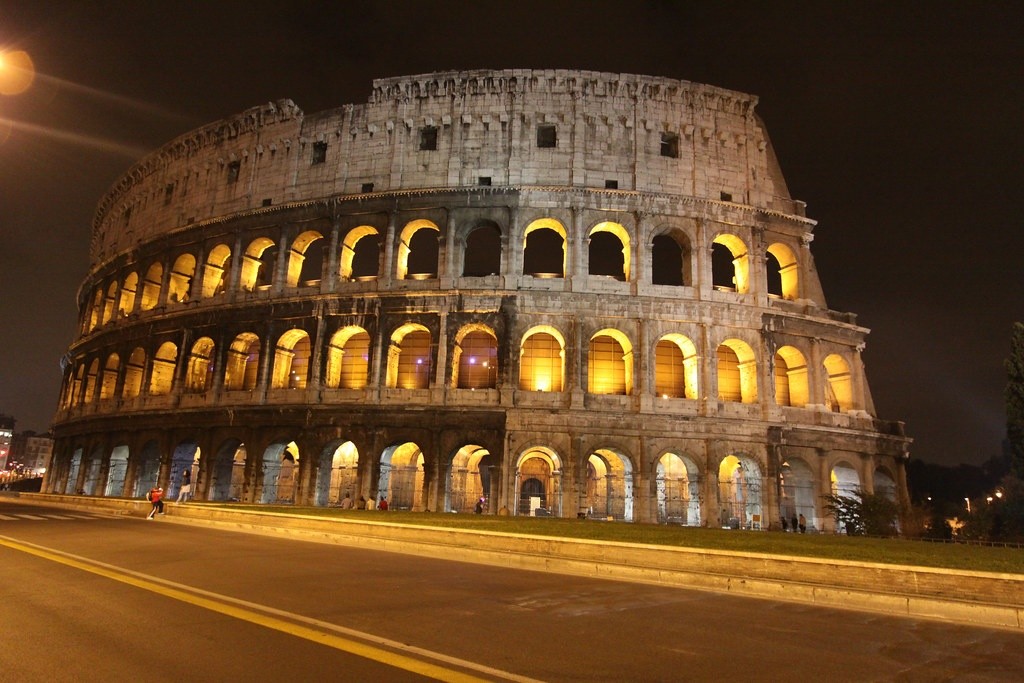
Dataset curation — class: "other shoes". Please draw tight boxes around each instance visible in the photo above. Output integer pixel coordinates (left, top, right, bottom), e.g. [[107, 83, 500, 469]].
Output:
[[146, 517, 153, 520], [157, 512, 164, 515]]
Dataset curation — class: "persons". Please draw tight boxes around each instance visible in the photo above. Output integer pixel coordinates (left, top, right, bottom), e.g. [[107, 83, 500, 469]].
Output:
[[146, 487, 165, 521], [342, 495, 388, 511], [476, 500, 487, 514], [176, 470, 191, 502], [781, 513, 807, 533]]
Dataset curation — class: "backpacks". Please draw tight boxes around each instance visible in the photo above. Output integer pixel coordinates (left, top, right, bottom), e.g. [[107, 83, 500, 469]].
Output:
[[145, 490, 151, 502]]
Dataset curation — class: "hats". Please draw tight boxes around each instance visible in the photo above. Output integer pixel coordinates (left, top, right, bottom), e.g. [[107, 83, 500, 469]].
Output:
[[158, 487, 164, 491]]
